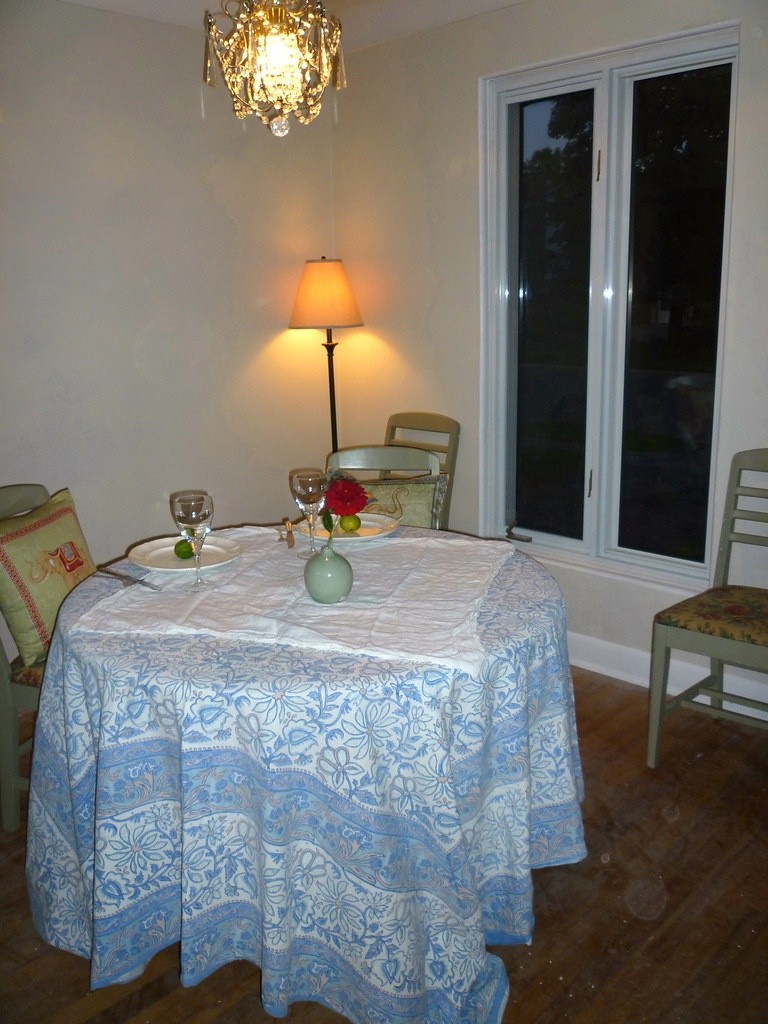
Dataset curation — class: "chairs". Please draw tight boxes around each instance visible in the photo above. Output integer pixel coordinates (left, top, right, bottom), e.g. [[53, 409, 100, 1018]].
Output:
[[647, 448, 768, 770], [0, 484, 48, 832], [326, 412, 461, 530]]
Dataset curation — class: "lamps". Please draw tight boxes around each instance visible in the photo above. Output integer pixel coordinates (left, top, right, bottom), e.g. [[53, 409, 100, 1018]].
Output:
[[205, 0, 347, 138], [287, 256, 364, 454]]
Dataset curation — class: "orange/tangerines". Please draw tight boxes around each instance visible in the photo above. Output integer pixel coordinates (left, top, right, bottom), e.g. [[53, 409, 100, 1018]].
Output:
[[340, 515, 360, 532], [174, 539, 195, 559]]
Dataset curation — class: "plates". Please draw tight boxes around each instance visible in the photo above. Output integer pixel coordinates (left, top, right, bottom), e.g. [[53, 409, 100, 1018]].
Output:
[[128, 535, 244, 571], [296, 512, 400, 542]]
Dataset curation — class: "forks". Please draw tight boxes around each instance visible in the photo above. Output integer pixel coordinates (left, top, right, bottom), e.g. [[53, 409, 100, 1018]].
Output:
[[92, 575, 145, 587], [278, 528, 286, 541]]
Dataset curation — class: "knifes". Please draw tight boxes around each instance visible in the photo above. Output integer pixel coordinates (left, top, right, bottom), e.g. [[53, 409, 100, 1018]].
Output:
[[97, 566, 162, 591], [285, 521, 295, 548]]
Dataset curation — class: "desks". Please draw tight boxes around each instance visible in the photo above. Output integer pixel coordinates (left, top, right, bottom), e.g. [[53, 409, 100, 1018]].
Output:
[[24, 525, 589, 1023]]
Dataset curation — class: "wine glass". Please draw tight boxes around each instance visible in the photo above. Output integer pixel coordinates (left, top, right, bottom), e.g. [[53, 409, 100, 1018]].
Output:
[[173, 495, 217, 591], [292, 474, 327, 560]]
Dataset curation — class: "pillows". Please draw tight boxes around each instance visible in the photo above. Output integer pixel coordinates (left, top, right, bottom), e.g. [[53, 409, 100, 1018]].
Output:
[[0, 487, 97, 667], [356, 474, 450, 530]]
